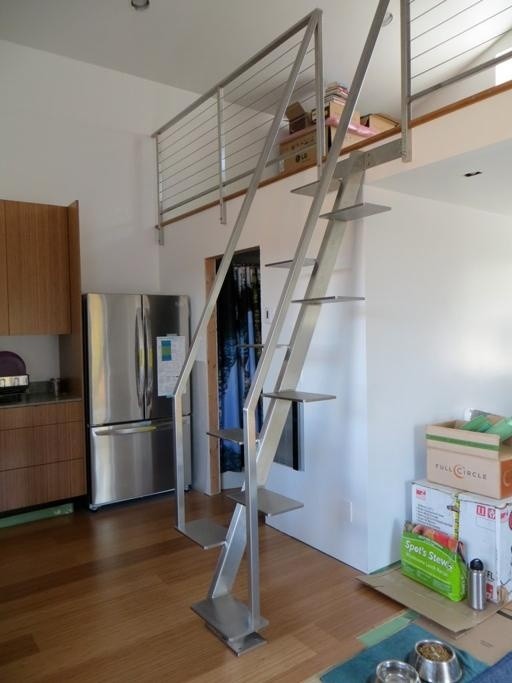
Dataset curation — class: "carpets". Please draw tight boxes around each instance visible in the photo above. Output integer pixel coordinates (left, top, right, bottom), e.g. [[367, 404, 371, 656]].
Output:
[[320, 624, 491, 683]]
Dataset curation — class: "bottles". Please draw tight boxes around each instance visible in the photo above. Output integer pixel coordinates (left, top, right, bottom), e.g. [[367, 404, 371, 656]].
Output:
[[467, 558, 488, 610]]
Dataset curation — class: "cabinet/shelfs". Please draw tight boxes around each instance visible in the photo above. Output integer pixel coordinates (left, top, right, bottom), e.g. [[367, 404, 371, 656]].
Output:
[[0, 199, 88, 529]]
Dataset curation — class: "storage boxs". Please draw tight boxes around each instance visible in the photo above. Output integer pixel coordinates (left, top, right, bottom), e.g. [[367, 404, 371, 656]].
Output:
[[412, 420, 512, 604], [278, 101, 399, 173]]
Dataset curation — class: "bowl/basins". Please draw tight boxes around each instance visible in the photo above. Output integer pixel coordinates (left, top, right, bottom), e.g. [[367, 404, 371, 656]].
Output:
[[407, 639, 463, 683], [370, 659, 422, 683]]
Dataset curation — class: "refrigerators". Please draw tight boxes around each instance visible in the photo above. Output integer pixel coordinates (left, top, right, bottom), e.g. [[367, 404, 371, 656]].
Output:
[[82, 290, 194, 511]]
